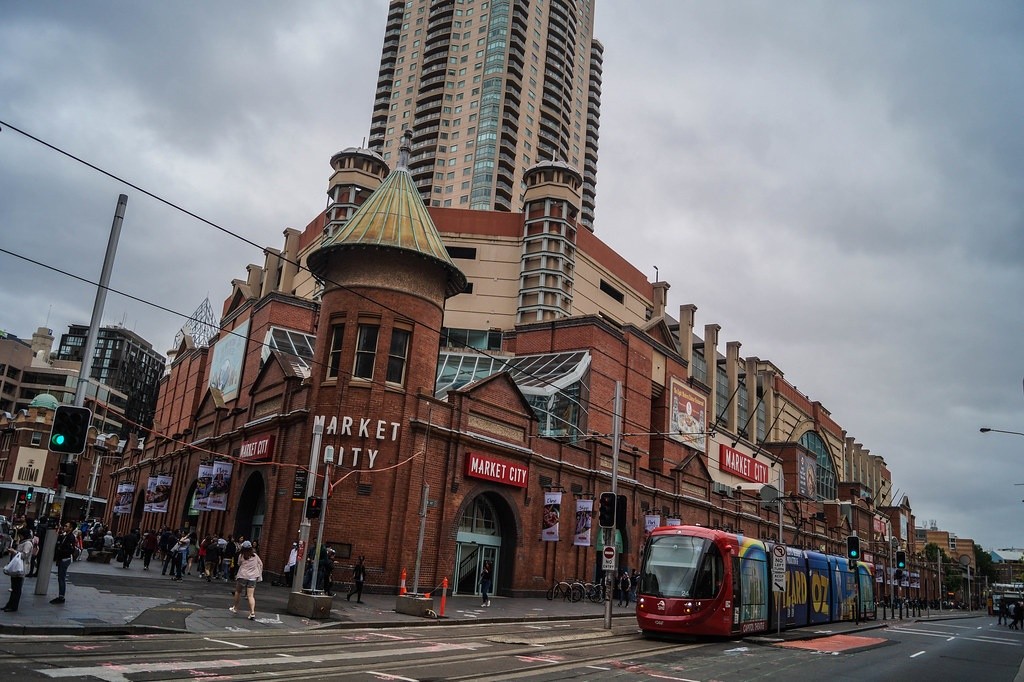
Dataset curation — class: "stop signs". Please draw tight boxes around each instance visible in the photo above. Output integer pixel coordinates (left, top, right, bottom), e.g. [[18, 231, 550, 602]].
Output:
[[603, 547, 615, 559]]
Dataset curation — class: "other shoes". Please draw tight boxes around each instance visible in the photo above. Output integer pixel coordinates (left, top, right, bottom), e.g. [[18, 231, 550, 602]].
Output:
[[123, 565, 130, 569], [8, 589, 13, 592], [144, 566, 149, 570], [346, 593, 351, 601], [480, 603, 486, 607], [229, 606, 239, 614], [50, 596, 65, 604], [487, 600, 491, 607], [25, 573, 33, 577], [1, 607, 17, 612], [248, 613, 255, 620], [357, 600, 363, 604], [161, 570, 229, 583], [33, 574, 38, 577]]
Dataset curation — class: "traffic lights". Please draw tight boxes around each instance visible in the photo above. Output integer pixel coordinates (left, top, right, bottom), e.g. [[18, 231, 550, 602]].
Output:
[[598, 492, 616, 527], [306, 496, 321, 520], [895, 551, 906, 569], [26, 487, 34, 500], [846, 536, 860, 560], [48, 405, 90, 455], [57, 461, 78, 487]]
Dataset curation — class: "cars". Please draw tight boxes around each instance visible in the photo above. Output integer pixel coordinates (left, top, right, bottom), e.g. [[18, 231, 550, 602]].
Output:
[[0, 515, 14, 556]]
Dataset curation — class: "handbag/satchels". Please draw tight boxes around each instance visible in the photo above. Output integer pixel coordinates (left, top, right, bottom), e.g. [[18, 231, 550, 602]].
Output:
[[230, 557, 235, 569], [4, 552, 24, 576], [117, 548, 124, 562], [284, 565, 290, 573], [255, 553, 263, 583]]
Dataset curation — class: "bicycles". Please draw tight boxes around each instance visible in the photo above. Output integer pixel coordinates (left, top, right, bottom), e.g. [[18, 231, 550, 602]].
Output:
[[133, 546, 160, 560], [546, 579, 606, 604]]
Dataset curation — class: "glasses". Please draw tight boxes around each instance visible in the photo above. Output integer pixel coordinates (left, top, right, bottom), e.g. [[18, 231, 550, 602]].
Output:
[[65, 524, 70, 527]]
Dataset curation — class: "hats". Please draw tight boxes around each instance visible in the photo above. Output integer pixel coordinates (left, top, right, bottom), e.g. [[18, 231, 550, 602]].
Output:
[[241, 540, 251, 548], [107, 531, 111, 534]]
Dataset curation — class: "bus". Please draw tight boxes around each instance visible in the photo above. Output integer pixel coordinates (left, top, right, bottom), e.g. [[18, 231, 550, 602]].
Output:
[[635, 521, 876, 635]]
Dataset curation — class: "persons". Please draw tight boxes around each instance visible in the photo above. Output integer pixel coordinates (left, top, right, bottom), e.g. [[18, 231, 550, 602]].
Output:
[[478, 564, 490, 607], [996, 599, 1024, 630], [0, 528, 33, 612], [347, 557, 367, 605], [893, 595, 927, 611], [600, 568, 640, 608], [228, 541, 263, 620], [9, 514, 259, 583], [49, 520, 78, 604], [285, 542, 339, 596]]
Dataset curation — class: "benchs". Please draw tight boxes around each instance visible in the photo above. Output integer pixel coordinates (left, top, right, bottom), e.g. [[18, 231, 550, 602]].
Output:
[[87, 550, 113, 564], [103, 546, 123, 560]]
